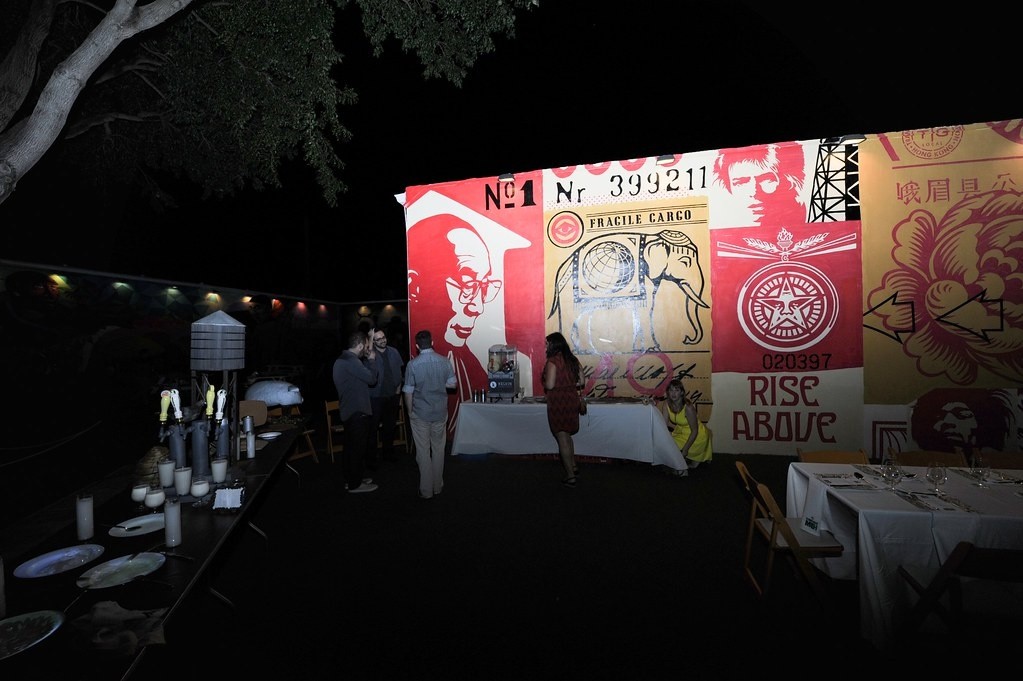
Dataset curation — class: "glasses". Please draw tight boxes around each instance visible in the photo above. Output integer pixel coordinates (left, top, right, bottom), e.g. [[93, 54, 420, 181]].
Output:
[[374, 336, 386, 341]]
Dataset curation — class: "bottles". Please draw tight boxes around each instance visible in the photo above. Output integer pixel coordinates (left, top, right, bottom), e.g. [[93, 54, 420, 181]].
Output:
[[487, 390, 490, 403], [473, 389, 478, 402], [480, 389, 485, 403], [247, 432, 255, 458], [242, 416, 253, 434]]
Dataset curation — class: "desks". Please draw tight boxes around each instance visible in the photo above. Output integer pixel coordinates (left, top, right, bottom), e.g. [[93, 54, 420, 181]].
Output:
[[451, 398, 689, 471], [0, 414, 309, 681], [787, 461, 1023, 651]]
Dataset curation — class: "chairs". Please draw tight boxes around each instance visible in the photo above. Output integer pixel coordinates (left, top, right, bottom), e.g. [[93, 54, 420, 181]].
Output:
[[270, 397, 410, 463], [734, 444, 1023, 610]]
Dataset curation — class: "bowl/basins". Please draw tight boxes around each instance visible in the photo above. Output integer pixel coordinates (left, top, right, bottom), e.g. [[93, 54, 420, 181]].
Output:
[[524, 397, 533, 402], [536, 398, 544, 401]]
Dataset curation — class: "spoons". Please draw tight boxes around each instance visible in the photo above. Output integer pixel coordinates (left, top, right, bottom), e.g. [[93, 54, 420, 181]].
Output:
[[903, 472, 918, 479], [853, 472, 879, 488], [102, 524, 142, 532]]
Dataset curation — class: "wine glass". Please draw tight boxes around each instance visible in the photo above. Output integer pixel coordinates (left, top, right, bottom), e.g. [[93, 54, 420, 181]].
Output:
[[145, 490, 166, 514], [190, 480, 210, 508], [883, 461, 904, 493], [132, 485, 151, 511], [927, 462, 948, 494], [971, 459, 989, 488]]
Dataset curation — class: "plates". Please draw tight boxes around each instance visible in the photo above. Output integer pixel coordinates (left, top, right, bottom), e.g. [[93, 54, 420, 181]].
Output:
[[257, 432, 281, 439], [14, 544, 105, 578], [76, 553, 165, 589], [109, 513, 165, 537], [0, 611, 63, 660]]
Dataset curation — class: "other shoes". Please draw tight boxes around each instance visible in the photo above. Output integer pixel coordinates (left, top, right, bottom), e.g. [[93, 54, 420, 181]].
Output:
[[572, 466, 578, 474], [383, 456, 398, 462], [417, 488, 433, 499], [560, 477, 576, 488], [434, 479, 443, 494]]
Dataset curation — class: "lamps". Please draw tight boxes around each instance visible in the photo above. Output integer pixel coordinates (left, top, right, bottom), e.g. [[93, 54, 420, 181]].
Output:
[[657, 155, 674, 165], [840, 134, 867, 145], [499, 172, 514, 182]]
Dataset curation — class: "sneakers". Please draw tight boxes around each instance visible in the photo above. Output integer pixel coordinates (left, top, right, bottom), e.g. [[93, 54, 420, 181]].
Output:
[[345, 478, 372, 489], [349, 483, 377, 492]]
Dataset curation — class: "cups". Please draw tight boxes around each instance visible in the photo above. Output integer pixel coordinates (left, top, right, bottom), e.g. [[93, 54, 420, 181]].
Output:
[[363, 350, 371, 357], [76, 495, 93, 540], [158, 459, 175, 489], [164, 498, 182, 547], [174, 467, 192, 498], [211, 457, 228, 484], [642, 397, 649, 405]]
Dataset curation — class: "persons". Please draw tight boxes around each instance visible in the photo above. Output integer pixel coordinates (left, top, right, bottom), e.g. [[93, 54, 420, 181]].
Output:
[[402, 331, 457, 499], [662, 379, 713, 468], [541, 332, 581, 488], [333, 327, 403, 493]]
